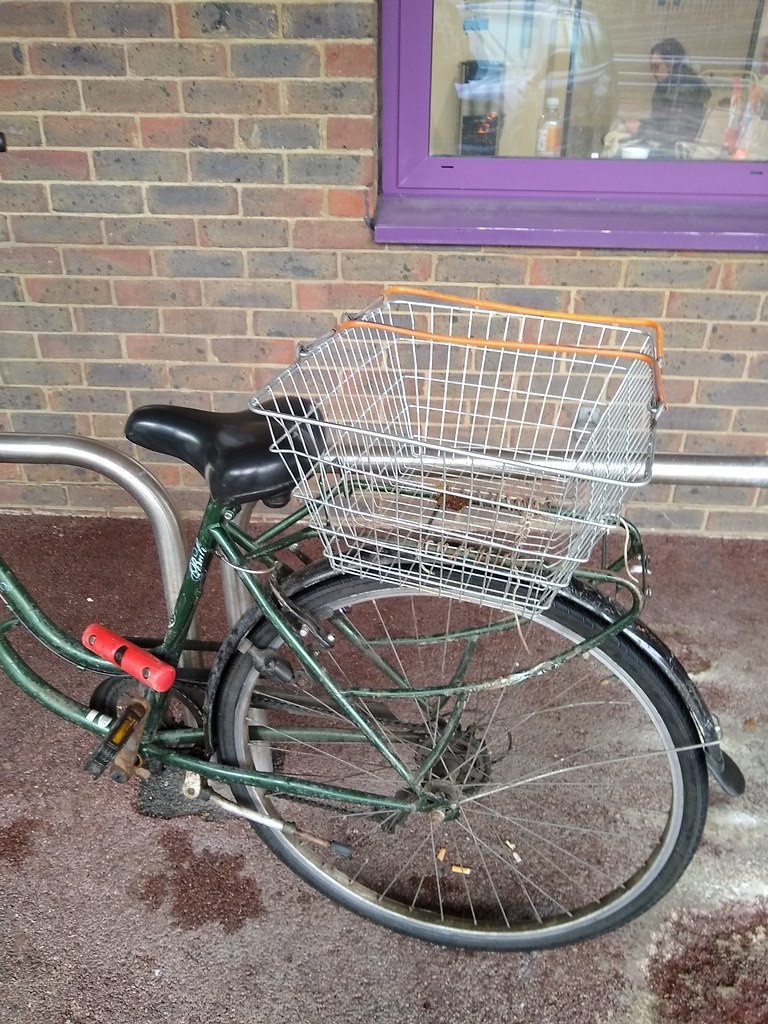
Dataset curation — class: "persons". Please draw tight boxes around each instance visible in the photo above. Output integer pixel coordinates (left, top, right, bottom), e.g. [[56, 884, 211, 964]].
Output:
[[614, 37, 711, 159]]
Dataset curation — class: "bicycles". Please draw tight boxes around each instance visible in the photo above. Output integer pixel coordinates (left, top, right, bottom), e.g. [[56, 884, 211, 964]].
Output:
[[0, 384, 748, 953]]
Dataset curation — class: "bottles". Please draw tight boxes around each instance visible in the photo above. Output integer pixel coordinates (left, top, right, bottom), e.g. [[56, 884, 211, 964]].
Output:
[[535, 96, 564, 159]]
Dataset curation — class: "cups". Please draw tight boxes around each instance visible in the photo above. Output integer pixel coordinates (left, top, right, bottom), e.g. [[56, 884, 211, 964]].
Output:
[[620, 147, 651, 159]]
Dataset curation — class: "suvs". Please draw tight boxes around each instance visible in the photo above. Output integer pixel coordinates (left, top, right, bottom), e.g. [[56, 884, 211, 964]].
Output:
[[456, 5, 617, 159]]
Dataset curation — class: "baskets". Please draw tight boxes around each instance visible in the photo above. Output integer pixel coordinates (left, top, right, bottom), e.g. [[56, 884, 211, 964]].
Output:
[[257, 288, 661, 616]]
